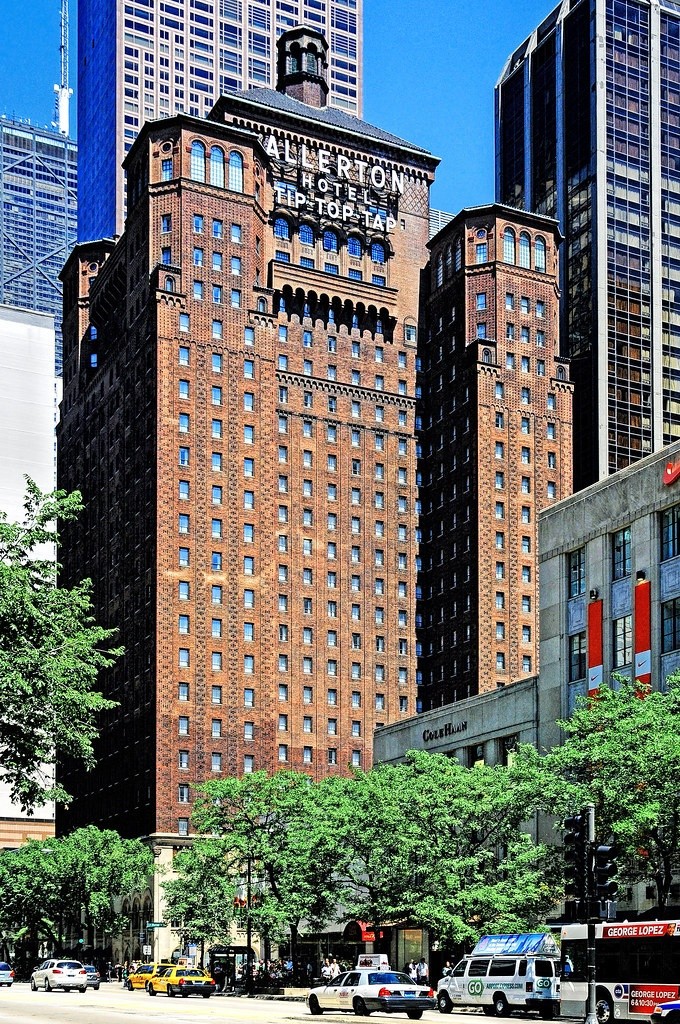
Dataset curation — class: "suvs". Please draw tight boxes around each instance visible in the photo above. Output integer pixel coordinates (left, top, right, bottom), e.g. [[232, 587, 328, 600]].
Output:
[[30, 958, 88, 993], [437, 933, 561, 1019]]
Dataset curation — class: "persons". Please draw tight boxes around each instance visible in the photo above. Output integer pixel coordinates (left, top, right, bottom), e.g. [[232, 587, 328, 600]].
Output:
[[238, 958, 313, 980], [442, 961, 452, 977], [402, 958, 428, 986], [214, 962, 225, 975], [321, 958, 352, 986], [116, 959, 146, 979]]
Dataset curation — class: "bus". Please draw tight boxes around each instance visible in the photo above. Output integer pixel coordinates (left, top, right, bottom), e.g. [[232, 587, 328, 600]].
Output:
[[557, 918, 680, 1023]]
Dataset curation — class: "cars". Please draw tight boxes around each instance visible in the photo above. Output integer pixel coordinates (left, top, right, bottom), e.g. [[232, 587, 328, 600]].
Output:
[[649, 1000, 680, 1024], [82, 965, 101, 990], [148, 957, 216, 998], [307, 954, 438, 1020], [123, 958, 181, 992], [0, 962, 15, 987]]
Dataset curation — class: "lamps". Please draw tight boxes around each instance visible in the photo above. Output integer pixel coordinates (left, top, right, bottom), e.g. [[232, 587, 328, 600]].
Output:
[[590, 589, 599, 600], [636, 570, 645, 582]]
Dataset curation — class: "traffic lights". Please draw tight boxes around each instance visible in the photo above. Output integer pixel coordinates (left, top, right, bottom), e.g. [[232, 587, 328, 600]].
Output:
[[562, 815, 582, 896], [79, 931, 84, 950], [589, 841, 619, 899]]
